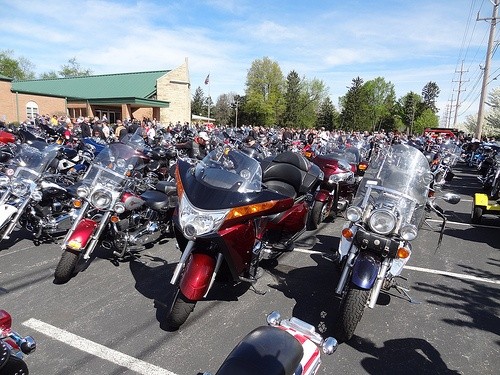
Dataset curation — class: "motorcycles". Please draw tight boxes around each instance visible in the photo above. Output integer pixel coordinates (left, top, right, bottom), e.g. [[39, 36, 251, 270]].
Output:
[[2, 118, 388, 186], [0, 141, 80, 245], [407, 135, 500, 199], [334, 142, 437, 340], [199, 310, 337, 375], [0, 308, 36, 375], [166, 148, 323, 331], [313, 146, 365, 226], [54, 143, 177, 284]]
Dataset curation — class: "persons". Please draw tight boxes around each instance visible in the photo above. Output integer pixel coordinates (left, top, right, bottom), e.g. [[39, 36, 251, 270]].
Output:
[[0, 113, 500, 170]]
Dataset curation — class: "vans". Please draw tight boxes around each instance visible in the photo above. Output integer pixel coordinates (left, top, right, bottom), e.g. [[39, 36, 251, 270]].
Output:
[[424, 128, 460, 146]]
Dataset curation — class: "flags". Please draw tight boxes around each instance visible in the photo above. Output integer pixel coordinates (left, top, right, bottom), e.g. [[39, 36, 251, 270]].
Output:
[[205, 74, 209, 85]]
